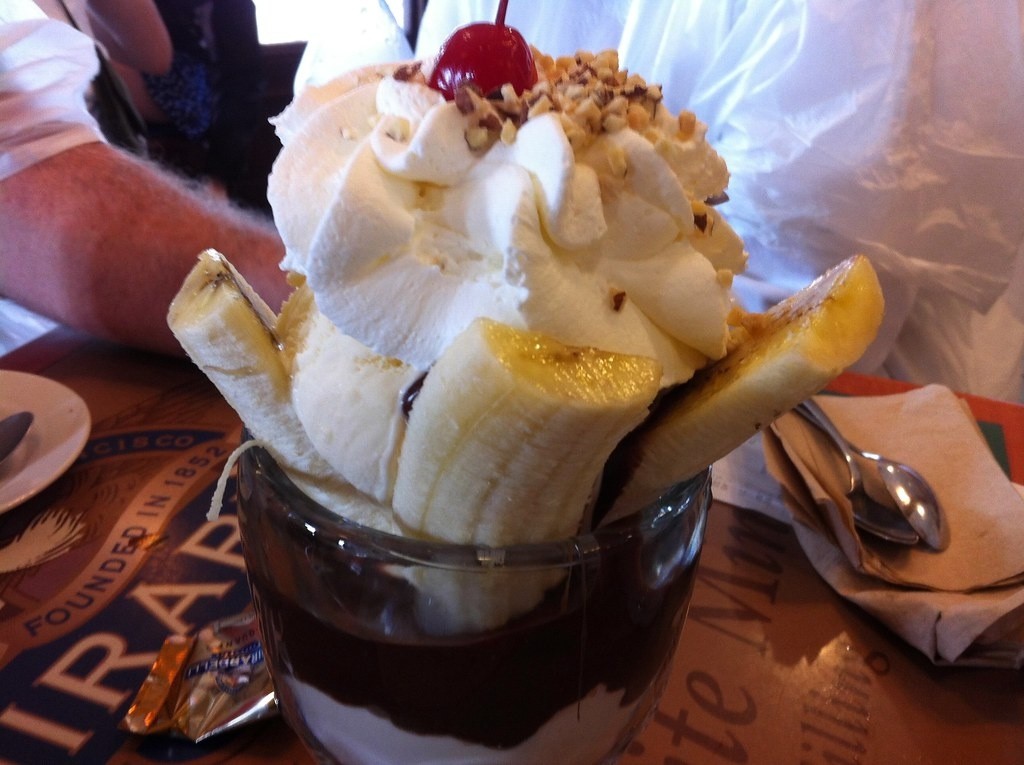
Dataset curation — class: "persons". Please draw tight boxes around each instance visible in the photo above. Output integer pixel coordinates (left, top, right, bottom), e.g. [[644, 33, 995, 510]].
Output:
[[0, 0, 309, 360]]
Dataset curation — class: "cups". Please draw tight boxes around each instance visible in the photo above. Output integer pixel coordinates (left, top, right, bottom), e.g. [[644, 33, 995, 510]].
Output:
[[236, 424, 714, 763]]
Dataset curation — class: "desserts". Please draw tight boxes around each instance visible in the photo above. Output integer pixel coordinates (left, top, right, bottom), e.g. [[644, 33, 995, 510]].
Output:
[[161, 0, 883, 765]]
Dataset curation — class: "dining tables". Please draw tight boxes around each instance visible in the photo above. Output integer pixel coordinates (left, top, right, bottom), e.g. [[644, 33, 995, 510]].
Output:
[[0, 322, 1022, 764]]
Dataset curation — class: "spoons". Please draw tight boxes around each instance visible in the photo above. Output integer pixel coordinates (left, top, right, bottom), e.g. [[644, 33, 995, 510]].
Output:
[[0, 412, 34, 466], [803, 394, 919, 545], [793, 404, 950, 550]]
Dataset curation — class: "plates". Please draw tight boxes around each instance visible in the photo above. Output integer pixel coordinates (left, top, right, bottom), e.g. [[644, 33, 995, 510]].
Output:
[[0, 369, 93, 516]]
